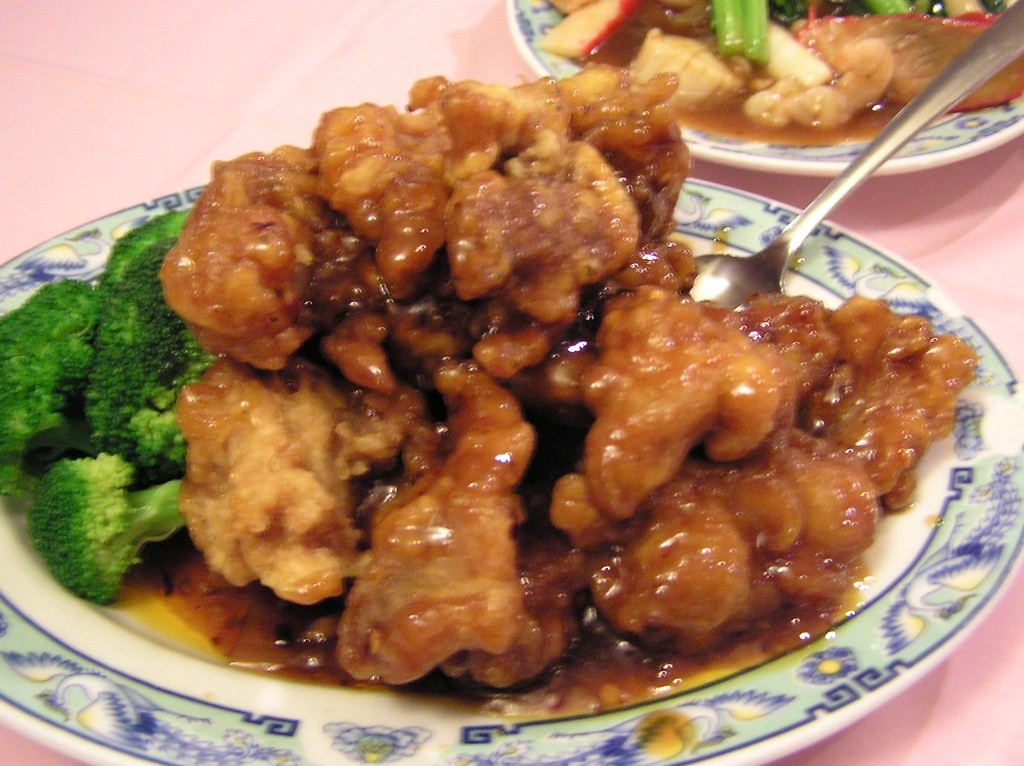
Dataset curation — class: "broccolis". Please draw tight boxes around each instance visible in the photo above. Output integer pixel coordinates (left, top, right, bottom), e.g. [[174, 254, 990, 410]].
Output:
[[0, 205, 231, 605]]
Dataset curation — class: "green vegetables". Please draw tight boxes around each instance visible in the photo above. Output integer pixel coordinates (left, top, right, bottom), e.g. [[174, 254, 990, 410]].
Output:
[[710, 0, 1009, 64]]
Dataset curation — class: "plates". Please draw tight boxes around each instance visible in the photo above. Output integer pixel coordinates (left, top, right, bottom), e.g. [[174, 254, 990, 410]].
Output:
[[505, 1, 1024, 177], [0, 179, 1023, 765]]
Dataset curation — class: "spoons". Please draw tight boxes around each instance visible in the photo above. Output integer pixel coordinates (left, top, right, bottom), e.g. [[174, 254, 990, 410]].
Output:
[[690, 0, 1024, 312]]
